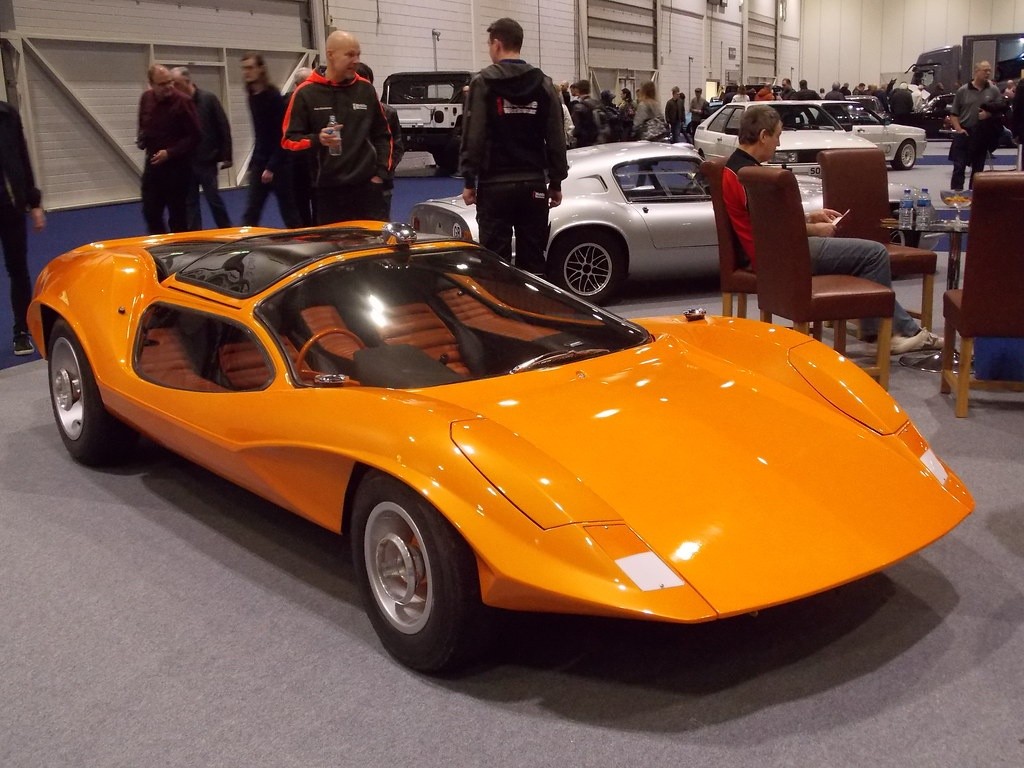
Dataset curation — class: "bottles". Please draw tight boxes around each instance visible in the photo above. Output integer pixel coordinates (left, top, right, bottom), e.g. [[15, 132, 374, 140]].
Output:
[[916, 188, 931, 231], [327, 116, 341, 156], [899, 189, 914, 230]]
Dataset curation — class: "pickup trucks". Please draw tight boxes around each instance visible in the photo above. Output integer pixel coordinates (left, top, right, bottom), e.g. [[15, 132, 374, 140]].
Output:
[[378, 70, 480, 173]]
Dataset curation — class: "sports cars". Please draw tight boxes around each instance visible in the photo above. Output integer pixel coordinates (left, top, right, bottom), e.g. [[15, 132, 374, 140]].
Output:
[[408, 139, 948, 308], [25, 220, 975, 681]]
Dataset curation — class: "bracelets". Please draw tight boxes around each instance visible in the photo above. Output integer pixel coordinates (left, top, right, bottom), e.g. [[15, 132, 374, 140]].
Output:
[[805, 212, 810, 223]]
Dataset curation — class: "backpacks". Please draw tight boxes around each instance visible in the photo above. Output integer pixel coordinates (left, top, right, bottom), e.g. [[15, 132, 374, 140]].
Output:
[[578, 100, 611, 138]]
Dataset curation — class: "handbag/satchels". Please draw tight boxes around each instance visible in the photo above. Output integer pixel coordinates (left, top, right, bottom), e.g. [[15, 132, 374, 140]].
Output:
[[636, 98, 669, 142]]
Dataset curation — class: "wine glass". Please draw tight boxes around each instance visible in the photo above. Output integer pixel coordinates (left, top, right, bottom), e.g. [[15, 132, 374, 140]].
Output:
[[940, 190, 973, 232]]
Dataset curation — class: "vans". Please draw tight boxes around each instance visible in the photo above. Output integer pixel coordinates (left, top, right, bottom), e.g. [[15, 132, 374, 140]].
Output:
[[724, 84, 784, 101]]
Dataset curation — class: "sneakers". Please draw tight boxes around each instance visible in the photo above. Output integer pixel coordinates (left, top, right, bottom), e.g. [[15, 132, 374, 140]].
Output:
[[862, 325, 947, 355], [13, 330, 34, 355]]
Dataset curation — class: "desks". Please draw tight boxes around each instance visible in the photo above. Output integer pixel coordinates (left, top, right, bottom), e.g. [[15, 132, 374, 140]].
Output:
[[880, 219, 976, 373]]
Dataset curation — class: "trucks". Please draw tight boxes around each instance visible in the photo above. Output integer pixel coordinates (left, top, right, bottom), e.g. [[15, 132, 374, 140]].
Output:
[[904, 33, 1024, 104]]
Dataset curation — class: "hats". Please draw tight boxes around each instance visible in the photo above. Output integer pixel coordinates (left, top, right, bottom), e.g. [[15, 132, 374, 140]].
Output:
[[900, 83, 907, 89], [918, 85, 923, 89]]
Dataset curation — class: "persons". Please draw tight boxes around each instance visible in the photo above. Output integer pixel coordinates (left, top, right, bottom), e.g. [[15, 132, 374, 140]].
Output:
[[459, 18, 569, 281], [716, 84, 783, 102], [779, 78, 796, 100], [818, 87, 826, 99], [886, 81, 913, 126], [852, 83, 865, 95], [839, 82, 851, 96], [242, 52, 314, 229], [0, 99, 48, 355], [1012, 77, 1024, 171], [912, 84, 924, 114], [555, 79, 664, 149], [789, 80, 823, 100], [135, 64, 233, 235], [1003, 79, 1017, 100], [690, 88, 707, 145], [863, 84, 877, 96], [953, 82, 961, 93], [722, 102, 948, 356], [823, 81, 848, 123], [929, 82, 945, 99], [665, 86, 691, 143], [874, 76, 897, 114], [947, 60, 1010, 191], [278, 30, 405, 226]]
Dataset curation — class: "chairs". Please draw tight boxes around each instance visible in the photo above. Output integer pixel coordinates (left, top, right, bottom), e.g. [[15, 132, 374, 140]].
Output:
[[782, 111, 804, 125], [818, 150, 937, 342], [941, 173, 1024, 418], [702, 160, 822, 340], [737, 167, 895, 392], [367, 303, 488, 377], [210, 316, 314, 388]]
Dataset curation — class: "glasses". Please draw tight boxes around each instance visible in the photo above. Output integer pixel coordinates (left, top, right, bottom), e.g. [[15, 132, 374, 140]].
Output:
[[151, 77, 174, 87]]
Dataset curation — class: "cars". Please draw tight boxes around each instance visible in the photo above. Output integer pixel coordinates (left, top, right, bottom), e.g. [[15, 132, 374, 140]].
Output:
[[681, 100, 723, 140], [694, 102, 878, 178], [795, 99, 928, 170], [843, 93, 895, 123], [904, 93, 957, 139]]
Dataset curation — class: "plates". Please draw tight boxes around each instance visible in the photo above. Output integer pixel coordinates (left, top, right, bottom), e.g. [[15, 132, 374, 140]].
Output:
[[934, 222, 969, 227], [943, 218, 970, 224]]
